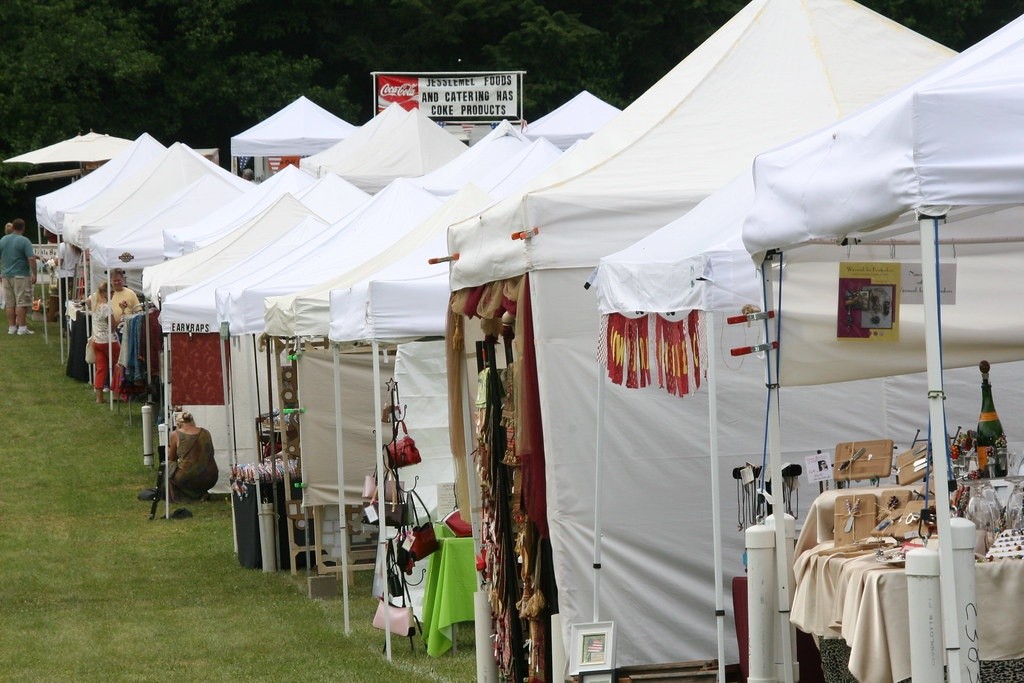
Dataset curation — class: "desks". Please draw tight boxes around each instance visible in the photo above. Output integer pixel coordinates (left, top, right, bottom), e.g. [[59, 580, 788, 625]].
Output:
[[421, 519, 477, 658]]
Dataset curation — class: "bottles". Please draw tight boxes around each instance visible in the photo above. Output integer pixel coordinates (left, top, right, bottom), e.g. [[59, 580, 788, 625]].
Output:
[[976, 360, 1008, 479]]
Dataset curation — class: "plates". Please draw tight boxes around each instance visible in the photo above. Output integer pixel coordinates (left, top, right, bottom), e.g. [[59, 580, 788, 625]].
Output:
[[875, 547, 905, 568]]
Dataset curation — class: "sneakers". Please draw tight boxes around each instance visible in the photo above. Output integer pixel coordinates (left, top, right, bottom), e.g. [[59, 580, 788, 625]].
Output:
[[8, 326, 18, 334], [17, 328, 35, 335]]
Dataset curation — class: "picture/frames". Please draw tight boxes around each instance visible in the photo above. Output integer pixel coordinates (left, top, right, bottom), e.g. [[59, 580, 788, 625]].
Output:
[[569, 621, 617, 672]]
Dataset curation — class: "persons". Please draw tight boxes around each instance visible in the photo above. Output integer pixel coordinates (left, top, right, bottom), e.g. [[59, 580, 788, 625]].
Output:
[[241, 169, 256, 185], [0, 219, 37, 335], [54, 236, 82, 337], [92, 282, 126, 404], [85, 268, 142, 392], [168, 411, 219, 503], [44, 228, 64, 244]]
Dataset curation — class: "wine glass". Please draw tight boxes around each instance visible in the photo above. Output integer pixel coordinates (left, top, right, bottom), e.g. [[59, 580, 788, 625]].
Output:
[[963, 481, 994, 556], [1005, 476, 1024, 530], [981, 480, 1001, 535]]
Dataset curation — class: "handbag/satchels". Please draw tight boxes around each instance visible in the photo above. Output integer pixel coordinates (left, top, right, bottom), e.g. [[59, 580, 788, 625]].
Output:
[[381, 381, 402, 423], [85, 336, 97, 363], [386, 420, 422, 468], [360, 455, 415, 527], [410, 490, 439, 561], [169, 460, 177, 478], [372, 562, 416, 637], [386, 539, 402, 597], [441, 505, 473, 538]]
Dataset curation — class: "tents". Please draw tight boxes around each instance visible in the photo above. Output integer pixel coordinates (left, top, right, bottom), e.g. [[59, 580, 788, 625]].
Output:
[[3, 0, 1024, 683]]
